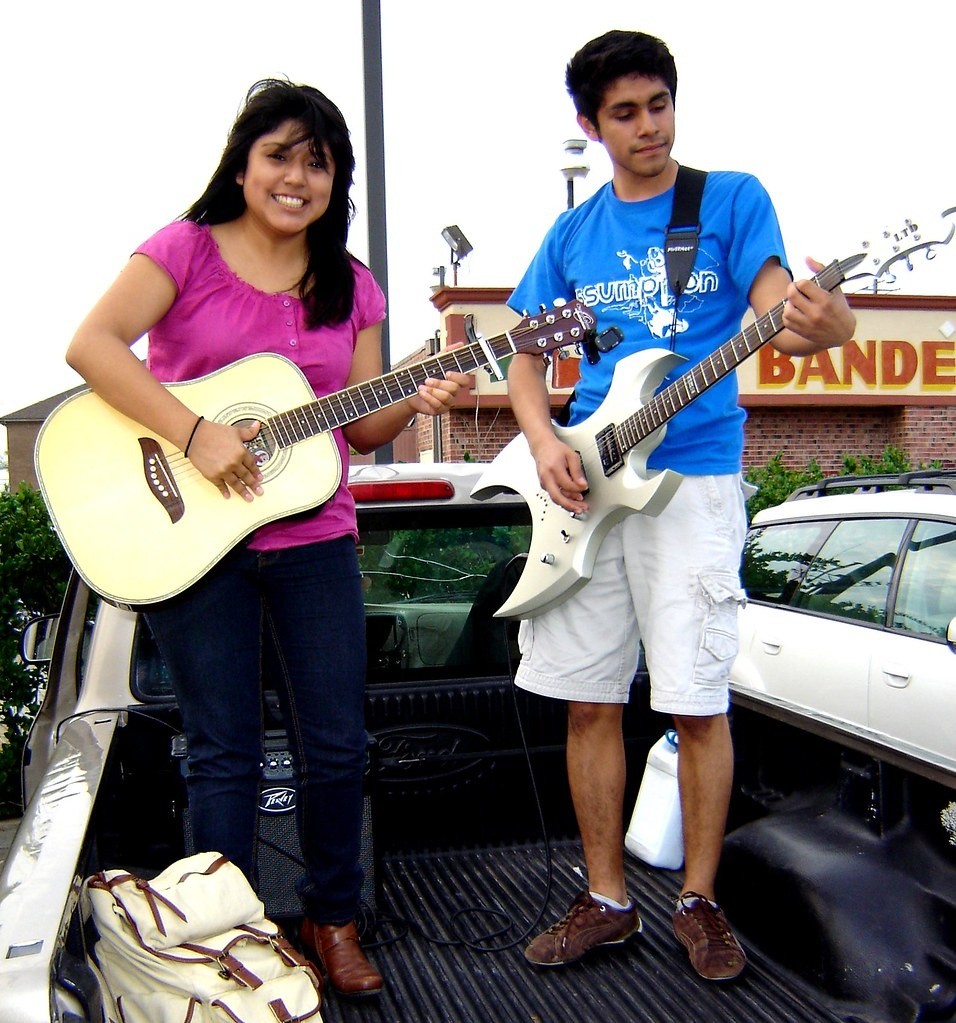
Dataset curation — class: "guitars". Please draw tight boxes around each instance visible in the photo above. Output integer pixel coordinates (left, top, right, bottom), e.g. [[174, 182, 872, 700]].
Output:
[[470, 201, 956, 618], [32, 298, 625, 611]]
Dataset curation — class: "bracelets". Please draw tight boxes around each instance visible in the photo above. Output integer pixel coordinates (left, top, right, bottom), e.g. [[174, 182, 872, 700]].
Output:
[[184, 416, 205, 459]]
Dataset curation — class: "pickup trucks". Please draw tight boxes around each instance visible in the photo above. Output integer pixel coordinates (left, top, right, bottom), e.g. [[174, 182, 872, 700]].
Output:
[[0, 458, 956, 1023]]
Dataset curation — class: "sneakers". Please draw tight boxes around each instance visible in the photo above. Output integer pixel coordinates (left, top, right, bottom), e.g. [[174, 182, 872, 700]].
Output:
[[526, 890, 642, 966], [673, 890, 745, 981]]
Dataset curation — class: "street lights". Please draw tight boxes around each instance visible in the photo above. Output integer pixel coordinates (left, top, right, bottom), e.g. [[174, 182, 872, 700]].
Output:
[[562, 164, 591, 209]]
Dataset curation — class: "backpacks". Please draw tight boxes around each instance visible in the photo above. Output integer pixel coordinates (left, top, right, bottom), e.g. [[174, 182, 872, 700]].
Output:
[[79, 851, 324, 1023]]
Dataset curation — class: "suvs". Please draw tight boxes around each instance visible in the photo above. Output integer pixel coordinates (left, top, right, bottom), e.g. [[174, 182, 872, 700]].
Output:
[[729, 469, 955, 772]]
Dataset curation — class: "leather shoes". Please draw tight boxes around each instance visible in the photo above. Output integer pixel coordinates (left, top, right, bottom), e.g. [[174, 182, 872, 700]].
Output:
[[299, 918, 382, 996]]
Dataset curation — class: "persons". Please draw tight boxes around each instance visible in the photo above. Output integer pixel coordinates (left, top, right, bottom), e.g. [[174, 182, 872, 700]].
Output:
[[65, 77, 467, 995], [508, 30, 855, 980]]
